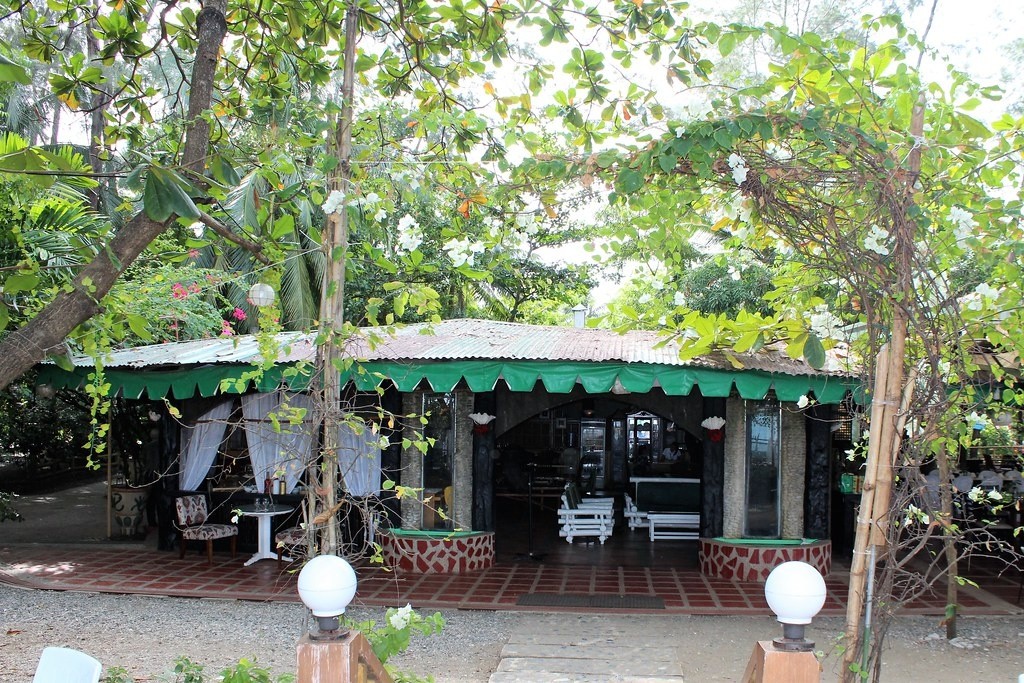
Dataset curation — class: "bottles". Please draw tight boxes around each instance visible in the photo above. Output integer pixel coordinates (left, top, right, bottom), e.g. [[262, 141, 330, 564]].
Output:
[[279, 475, 286, 495], [272, 476, 280, 495], [264, 472, 272, 494]]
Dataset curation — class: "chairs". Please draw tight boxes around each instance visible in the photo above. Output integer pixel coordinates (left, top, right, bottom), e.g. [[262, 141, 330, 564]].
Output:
[[557, 486, 615, 544], [564, 481, 615, 525], [274, 500, 327, 567], [913, 467, 1024, 530], [175, 494, 238, 565]]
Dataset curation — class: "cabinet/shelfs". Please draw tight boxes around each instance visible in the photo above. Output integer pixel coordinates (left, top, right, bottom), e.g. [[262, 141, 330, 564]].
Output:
[[628, 417, 663, 463]]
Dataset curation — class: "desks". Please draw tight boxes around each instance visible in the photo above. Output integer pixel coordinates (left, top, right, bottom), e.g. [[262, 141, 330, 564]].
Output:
[[233, 503, 295, 567], [646, 511, 700, 542], [422, 488, 445, 530]]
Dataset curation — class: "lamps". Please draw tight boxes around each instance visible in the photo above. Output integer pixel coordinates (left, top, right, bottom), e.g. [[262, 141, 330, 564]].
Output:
[[249, 281, 275, 307], [467, 412, 498, 433], [764, 560, 828, 650], [297, 554, 357, 643], [700, 416, 727, 442]]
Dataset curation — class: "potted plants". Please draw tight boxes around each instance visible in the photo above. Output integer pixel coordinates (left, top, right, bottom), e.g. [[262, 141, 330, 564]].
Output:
[[106, 407, 149, 538]]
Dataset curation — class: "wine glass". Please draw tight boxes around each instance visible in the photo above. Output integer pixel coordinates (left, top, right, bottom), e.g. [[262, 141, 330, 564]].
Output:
[[262, 498, 270, 512], [253, 497, 262, 511]]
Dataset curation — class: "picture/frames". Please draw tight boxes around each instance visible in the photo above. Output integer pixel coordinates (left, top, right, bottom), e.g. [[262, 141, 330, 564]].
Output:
[[540, 410, 549, 418], [555, 417, 566, 429]]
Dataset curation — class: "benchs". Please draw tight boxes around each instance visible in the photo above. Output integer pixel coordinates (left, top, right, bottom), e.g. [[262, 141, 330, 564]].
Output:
[[624, 476, 701, 531]]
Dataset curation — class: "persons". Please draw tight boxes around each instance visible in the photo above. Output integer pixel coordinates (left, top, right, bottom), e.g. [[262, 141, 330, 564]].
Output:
[[557, 441, 692, 494]]
